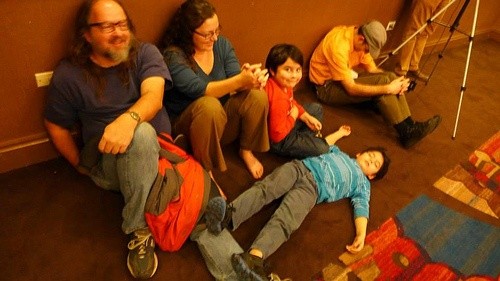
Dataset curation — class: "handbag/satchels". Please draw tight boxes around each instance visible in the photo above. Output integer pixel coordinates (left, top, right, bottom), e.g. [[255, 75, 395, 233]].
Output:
[[144, 132, 210, 253]]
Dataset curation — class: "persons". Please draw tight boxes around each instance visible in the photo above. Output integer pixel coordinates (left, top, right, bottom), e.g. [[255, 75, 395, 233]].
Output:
[[394, 0, 453, 82], [44, 0, 243, 281], [161, 0, 271, 200], [264, 43, 328, 156], [204, 145, 390, 281], [308, 21, 442, 149]]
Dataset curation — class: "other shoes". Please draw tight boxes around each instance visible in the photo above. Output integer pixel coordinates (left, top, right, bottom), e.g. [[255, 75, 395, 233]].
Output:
[[397, 114, 443, 149], [394, 64, 408, 75], [407, 70, 429, 82]]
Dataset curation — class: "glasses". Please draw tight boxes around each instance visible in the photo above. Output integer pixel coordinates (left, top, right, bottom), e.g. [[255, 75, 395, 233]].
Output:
[[88, 18, 130, 34], [194, 24, 223, 40]]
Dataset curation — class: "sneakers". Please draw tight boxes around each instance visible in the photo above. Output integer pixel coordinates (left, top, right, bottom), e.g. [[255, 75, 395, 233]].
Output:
[[204, 196, 235, 236], [127, 225, 159, 280], [232, 251, 270, 281]]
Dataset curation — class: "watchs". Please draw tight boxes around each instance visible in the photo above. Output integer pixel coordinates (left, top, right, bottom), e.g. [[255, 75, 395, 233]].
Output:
[[127, 111, 141, 125]]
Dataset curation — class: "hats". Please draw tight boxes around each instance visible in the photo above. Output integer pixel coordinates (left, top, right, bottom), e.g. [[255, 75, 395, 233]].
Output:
[[363, 20, 387, 59]]
